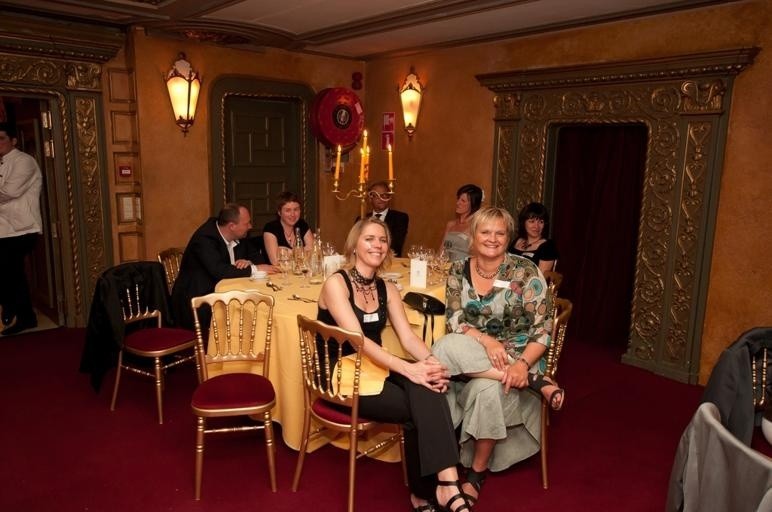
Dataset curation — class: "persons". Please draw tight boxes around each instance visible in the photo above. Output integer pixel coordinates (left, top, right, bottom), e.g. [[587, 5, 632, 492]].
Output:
[[508, 203, 556, 284], [354, 182, 409, 258], [430, 205, 565, 507], [260, 192, 315, 266], [435, 184, 482, 263], [312, 217, 473, 512], [0, 122, 43, 335], [170, 201, 282, 329]]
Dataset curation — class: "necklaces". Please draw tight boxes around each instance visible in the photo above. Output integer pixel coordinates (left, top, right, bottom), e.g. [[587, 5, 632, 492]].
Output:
[[350, 265, 377, 303], [521, 237, 543, 250], [283, 228, 294, 241], [475, 256, 504, 279]]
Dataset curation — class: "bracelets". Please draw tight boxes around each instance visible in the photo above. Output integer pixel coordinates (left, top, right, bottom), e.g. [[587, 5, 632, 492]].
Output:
[[517, 357, 530, 371], [476, 332, 487, 342], [423, 352, 433, 361]]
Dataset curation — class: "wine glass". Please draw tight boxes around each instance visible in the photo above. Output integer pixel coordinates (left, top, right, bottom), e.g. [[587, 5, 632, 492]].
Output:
[[409, 244, 448, 286], [277, 244, 337, 281]]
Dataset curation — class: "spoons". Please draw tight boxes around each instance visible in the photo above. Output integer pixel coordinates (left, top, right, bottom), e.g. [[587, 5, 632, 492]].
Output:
[[289, 294, 316, 305], [265, 281, 282, 293]]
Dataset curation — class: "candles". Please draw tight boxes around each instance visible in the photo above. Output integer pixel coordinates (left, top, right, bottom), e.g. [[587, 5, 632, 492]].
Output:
[[362, 129, 369, 152], [358, 148, 365, 183], [334, 144, 344, 180], [365, 145, 370, 165], [386, 143, 396, 179]]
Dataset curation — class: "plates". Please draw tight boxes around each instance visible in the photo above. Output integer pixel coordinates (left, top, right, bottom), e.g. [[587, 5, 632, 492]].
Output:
[[381, 270, 404, 295]]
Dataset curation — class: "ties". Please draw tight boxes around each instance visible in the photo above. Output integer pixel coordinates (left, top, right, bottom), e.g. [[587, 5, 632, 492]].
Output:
[[373, 212, 383, 221]]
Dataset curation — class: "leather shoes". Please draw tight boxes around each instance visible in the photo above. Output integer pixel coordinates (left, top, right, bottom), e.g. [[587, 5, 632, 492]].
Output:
[[0, 310, 40, 336]]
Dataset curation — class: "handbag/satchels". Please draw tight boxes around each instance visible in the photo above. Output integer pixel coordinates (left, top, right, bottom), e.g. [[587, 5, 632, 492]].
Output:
[[401, 290, 446, 317]]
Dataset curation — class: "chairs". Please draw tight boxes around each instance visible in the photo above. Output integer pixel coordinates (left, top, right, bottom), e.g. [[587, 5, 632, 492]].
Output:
[[537, 295, 571, 490], [699, 398, 772, 511], [540, 269, 562, 298], [292, 315, 412, 512], [552, 250, 561, 272], [749, 344, 770, 407], [155, 246, 187, 287], [106, 258, 206, 426], [189, 288, 279, 504]]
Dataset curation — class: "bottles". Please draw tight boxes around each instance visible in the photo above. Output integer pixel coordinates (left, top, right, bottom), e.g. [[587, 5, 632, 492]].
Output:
[[291, 227, 306, 275], [312, 228, 325, 278]]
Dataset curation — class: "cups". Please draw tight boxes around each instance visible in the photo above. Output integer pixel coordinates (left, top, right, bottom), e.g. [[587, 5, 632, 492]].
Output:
[[253, 270, 267, 278]]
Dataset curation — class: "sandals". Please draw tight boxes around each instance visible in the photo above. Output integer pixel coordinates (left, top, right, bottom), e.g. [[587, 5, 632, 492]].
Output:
[[408, 490, 438, 512], [434, 477, 475, 512], [460, 465, 489, 509], [525, 369, 567, 414]]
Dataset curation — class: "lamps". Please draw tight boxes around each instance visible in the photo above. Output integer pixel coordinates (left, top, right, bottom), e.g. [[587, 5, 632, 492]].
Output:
[[160, 51, 206, 137], [392, 65, 430, 145]]
